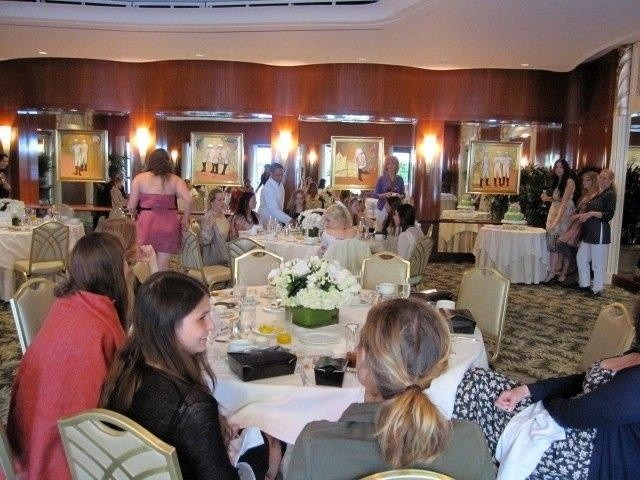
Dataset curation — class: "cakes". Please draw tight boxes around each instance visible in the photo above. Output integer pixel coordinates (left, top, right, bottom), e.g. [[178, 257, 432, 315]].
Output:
[[458, 194, 474, 207], [503, 202, 524, 221]]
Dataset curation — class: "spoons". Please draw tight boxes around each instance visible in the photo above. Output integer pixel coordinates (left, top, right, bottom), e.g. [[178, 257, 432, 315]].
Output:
[[214, 301, 235, 309]]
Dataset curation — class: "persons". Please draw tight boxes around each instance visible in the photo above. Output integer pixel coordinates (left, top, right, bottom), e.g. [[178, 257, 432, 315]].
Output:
[[94, 160, 129, 228], [541, 158, 616, 298], [255, 164, 270, 192], [473, 194, 509, 219], [1, 233, 129, 480], [454, 297, 639, 480], [2, 152, 11, 199], [280, 298, 496, 479], [200, 188, 259, 268], [258, 162, 296, 229], [225, 179, 254, 193], [283, 176, 424, 261], [128, 149, 192, 270], [94, 271, 269, 480], [374, 155, 406, 219], [184, 179, 198, 201], [442, 160, 455, 193]]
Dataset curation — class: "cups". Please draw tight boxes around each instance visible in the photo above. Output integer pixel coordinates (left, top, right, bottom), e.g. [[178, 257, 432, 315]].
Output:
[[255, 336, 269, 350], [375, 282, 395, 296], [228, 340, 258, 354], [351, 295, 360, 306], [344, 323, 361, 367], [266, 277, 276, 298], [435, 300, 456, 310], [0, 207, 81, 233], [246, 295, 255, 303], [274, 310, 295, 351], [257, 216, 387, 245], [229, 320, 242, 339]]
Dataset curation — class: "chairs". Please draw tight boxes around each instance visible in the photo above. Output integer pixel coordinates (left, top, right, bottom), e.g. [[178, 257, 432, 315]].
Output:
[[56, 410, 186, 479], [358, 467, 456, 480], [453, 266, 511, 366], [577, 301, 633, 370], [2, 194, 434, 294], [8, 277, 61, 358], [0, 419, 21, 480]]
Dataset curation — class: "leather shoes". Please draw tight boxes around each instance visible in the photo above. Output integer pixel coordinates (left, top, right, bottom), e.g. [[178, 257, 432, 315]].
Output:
[[585, 290, 601, 298], [570, 283, 591, 292], [542, 275, 559, 286]]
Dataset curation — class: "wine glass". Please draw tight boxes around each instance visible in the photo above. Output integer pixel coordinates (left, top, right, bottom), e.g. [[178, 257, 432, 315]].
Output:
[[541, 190, 547, 207], [232, 280, 247, 309], [240, 299, 257, 338]]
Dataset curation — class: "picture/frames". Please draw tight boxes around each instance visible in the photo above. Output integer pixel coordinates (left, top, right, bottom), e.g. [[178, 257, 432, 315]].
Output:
[[189, 132, 245, 189], [330, 135, 386, 191], [465, 141, 522, 196], [53, 127, 111, 183]]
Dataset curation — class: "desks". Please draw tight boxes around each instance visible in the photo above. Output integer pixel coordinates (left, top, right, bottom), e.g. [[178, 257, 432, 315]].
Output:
[[438, 206, 556, 288]]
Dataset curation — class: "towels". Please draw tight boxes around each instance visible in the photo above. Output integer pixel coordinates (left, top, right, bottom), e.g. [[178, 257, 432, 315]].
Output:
[[226, 421, 264, 467], [494, 399, 569, 480]]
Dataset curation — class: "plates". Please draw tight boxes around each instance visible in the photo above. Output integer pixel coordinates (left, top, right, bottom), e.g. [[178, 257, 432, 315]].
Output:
[[218, 308, 240, 322], [300, 331, 340, 345], [253, 326, 285, 336], [240, 299, 260, 306], [262, 303, 286, 314]]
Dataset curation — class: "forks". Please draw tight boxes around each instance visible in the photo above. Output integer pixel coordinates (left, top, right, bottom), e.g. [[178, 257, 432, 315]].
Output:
[[294, 358, 310, 386], [450, 335, 479, 342]]
